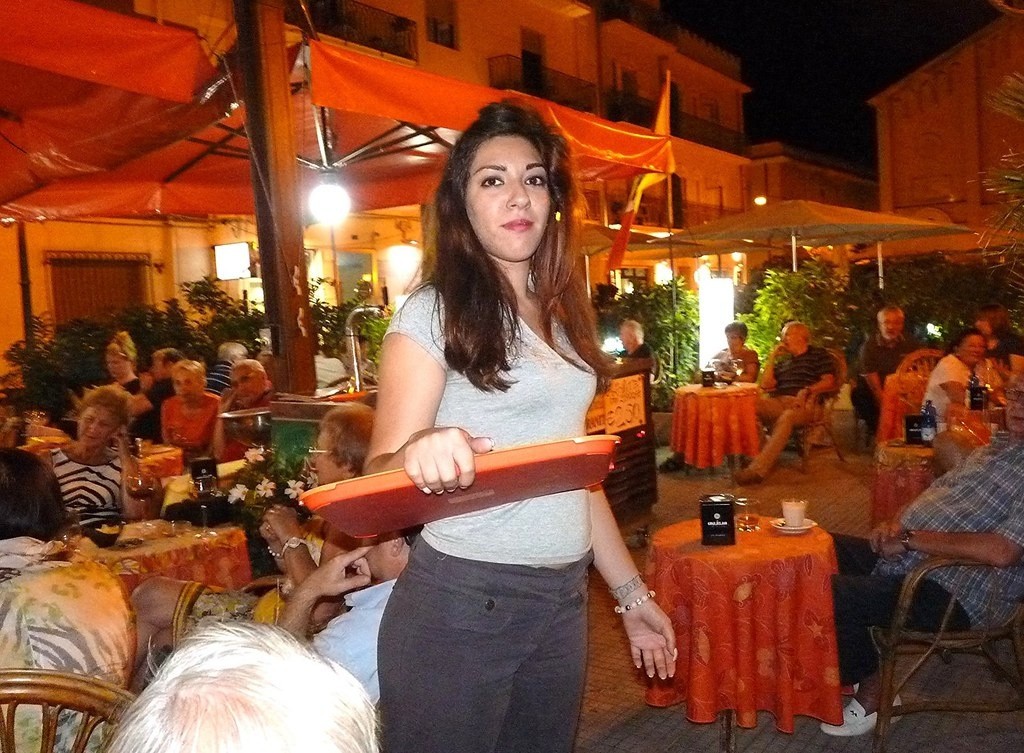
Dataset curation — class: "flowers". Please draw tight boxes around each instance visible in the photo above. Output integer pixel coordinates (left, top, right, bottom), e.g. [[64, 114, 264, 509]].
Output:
[[228, 446, 314, 527]]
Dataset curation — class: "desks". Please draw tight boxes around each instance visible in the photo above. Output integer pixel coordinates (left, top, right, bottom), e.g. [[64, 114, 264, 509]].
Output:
[[67, 520, 250, 618], [19, 434, 75, 457], [673, 383, 762, 482], [118, 440, 183, 492], [874, 439, 941, 535], [873, 369, 936, 443], [632, 516, 843, 753], [946, 400, 1005, 466]]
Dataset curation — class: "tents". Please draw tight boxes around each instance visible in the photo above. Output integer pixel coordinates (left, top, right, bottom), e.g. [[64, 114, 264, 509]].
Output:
[[1, 0, 667, 351]]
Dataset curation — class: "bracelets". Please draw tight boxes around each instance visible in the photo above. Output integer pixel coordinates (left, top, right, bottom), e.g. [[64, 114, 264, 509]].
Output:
[[614, 590, 656, 614], [606, 574, 644, 601], [267, 544, 284, 559]]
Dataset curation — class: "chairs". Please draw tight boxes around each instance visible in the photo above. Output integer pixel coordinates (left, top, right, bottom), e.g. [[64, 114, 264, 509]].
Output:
[[0, 350, 1024, 753]]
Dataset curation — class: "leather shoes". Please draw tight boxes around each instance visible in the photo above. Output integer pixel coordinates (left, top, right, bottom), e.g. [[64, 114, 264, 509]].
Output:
[[821, 694, 903, 737], [852, 682, 861, 693]]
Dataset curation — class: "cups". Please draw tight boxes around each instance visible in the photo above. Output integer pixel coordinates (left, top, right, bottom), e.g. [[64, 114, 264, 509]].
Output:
[[735, 498, 760, 531], [714, 381, 727, 387], [165, 520, 190, 537], [937, 423, 946, 434], [57, 515, 82, 551], [782, 502, 805, 527]]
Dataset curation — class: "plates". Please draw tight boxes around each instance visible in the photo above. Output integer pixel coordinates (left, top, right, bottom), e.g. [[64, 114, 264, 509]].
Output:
[[770, 519, 817, 534]]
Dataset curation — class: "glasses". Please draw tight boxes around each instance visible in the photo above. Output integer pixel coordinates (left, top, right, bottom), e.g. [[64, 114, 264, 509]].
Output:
[[309, 445, 330, 463], [229, 371, 258, 390]]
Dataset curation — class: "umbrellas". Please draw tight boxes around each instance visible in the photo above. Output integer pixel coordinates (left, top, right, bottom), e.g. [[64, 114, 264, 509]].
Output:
[[637, 197, 972, 288]]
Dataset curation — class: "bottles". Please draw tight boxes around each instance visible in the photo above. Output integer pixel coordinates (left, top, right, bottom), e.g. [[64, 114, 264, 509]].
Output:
[[921, 400, 936, 446]]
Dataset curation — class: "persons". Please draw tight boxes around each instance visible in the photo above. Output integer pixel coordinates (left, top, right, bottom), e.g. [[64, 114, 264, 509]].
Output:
[[0, 447, 137, 752], [851, 304, 919, 454], [363, 101, 678, 753], [705, 322, 759, 383], [96, 618, 379, 753], [128, 402, 425, 707], [26, 384, 134, 530], [920, 301, 1024, 421], [616, 320, 650, 360], [732, 320, 839, 487], [313, 333, 375, 388], [93, 330, 273, 457], [819, 366, 1024, 738]]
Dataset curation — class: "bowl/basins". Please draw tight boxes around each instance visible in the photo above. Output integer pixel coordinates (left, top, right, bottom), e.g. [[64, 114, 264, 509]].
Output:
[[84, 520, 125, 548], [217, 407, 272, 449]]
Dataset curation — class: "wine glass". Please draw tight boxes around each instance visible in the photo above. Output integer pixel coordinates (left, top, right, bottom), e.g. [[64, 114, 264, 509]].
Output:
[[125, 472, 158, 523], [188, 476, 221, 540]]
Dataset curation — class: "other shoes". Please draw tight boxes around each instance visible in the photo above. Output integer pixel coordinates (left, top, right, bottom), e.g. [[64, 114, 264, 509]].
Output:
[[733, 468, 764, 487]]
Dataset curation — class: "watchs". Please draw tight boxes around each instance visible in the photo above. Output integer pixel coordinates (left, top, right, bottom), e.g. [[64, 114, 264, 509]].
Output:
[[896, 528, 912, 552], [805, 384, 812, 395], [282, 537, 308, 552]]
